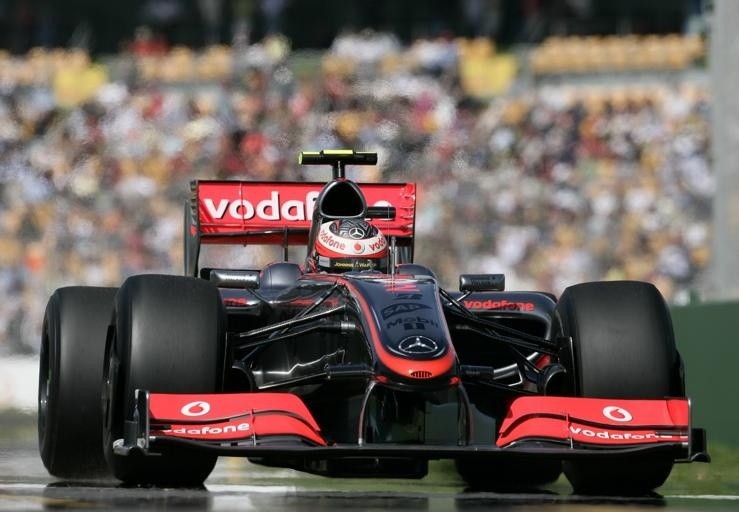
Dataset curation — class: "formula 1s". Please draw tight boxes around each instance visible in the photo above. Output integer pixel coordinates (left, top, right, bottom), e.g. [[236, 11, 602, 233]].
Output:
[[37, 149, 712, 494]]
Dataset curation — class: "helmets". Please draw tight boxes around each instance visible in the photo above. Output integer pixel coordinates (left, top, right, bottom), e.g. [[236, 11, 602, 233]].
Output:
[[308, 219, 390, 274]]
